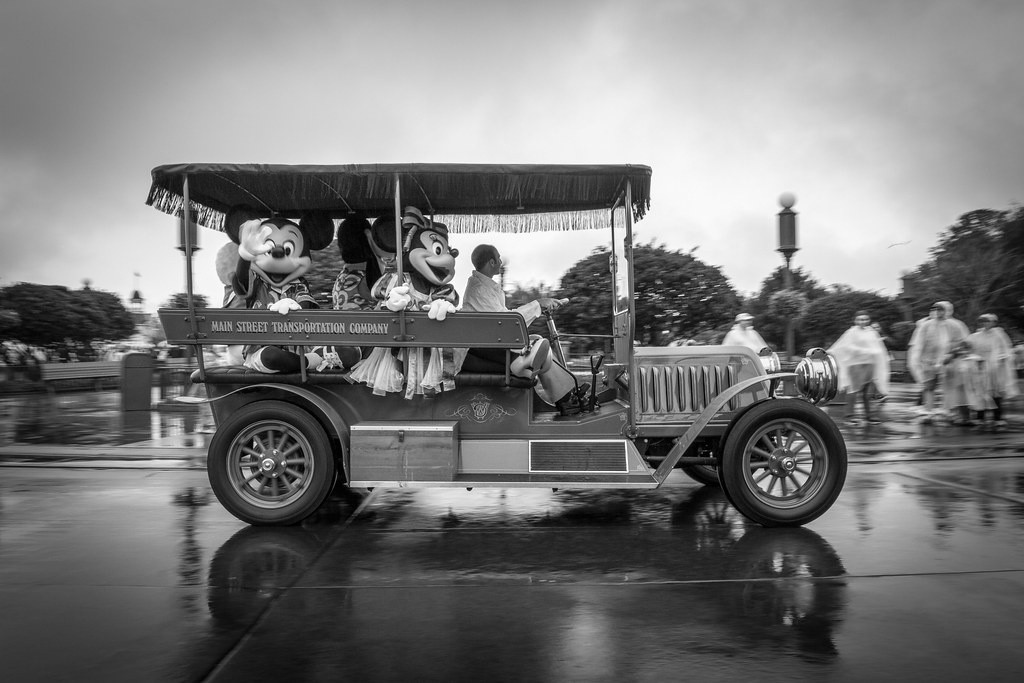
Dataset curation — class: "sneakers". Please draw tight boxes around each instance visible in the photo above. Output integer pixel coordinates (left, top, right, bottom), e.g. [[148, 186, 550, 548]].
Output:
[[572, 382, 590, 397], [560, 392, 591, 415]]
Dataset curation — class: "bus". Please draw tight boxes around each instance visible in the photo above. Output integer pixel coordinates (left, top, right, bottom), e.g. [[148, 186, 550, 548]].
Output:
[[145, 162, 848, 527]]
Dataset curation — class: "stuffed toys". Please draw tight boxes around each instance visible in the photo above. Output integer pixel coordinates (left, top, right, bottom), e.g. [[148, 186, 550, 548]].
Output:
[[216, 204, 549, 401]]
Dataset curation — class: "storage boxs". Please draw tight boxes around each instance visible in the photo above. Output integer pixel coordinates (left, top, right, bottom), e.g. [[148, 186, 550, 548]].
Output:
[[350, 420, 460, 481]]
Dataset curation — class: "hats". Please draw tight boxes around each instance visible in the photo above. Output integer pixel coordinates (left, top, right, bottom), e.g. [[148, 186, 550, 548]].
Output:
[[977, 312, 998, 322], [734, 313, 755, 322], [930, 302, 945, 311]]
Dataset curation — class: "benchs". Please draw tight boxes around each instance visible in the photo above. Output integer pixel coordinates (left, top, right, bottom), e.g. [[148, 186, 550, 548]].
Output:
[[191, 363, 536, 388], [40, 361, 120, 393]]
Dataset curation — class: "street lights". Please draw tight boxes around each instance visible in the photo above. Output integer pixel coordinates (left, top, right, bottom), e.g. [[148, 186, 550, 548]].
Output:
[[778, 191, 800, 397], [898, 265, 916, 383]]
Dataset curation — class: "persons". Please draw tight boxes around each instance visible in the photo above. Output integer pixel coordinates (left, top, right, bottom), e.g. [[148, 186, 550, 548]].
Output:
[[462, 244, 601, 415], [824, 310, 893, 426], [905, 302, 1022, 427], [722, 313, 773, 357]]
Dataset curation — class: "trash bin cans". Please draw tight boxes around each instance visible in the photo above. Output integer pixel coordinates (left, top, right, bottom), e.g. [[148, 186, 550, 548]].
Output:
[[633, 340, 641, 347], [168, 346, 187, 358], [122, 352, 153, 411], [550, 340, 572, 362]]
